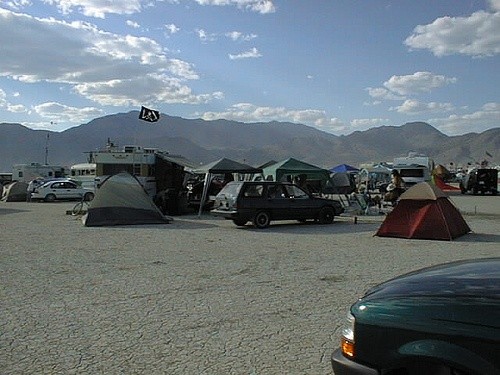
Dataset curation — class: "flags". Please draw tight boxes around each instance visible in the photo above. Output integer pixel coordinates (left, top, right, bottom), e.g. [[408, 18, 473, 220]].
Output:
[[139, 106, 159, 124]]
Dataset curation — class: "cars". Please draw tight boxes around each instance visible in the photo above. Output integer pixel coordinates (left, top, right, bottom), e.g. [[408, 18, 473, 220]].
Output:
[[209, 180, 345, 229], [330, 256, 500, 375], [30, 179, 95, 203]]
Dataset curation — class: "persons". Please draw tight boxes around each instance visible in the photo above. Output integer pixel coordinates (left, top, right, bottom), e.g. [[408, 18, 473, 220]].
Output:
[[391, 169, 406, 210], [364, 194, 385, 215]]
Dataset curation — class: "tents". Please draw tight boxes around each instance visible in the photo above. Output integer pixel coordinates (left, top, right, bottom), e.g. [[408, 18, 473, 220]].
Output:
[[372, 180, 472, 241], [251, 160, 278, 181], [6, 182, 31, 202], [178, 157, 265, 218], [81, 171, 169, 226], [329, 164, 360, 174], [263, 158, 329, 194]]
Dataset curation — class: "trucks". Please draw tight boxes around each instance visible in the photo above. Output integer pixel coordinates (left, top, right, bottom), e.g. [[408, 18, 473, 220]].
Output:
[[394, 157, 433, 188]]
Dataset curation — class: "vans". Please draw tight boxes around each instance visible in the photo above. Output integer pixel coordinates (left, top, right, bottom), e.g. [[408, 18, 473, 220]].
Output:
[[459, 168, 499, 196]]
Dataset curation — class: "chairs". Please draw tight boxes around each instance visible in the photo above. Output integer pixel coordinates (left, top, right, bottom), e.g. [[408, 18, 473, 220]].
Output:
[[247, 188, 260, 196], [354, 194, 376, 216]]
